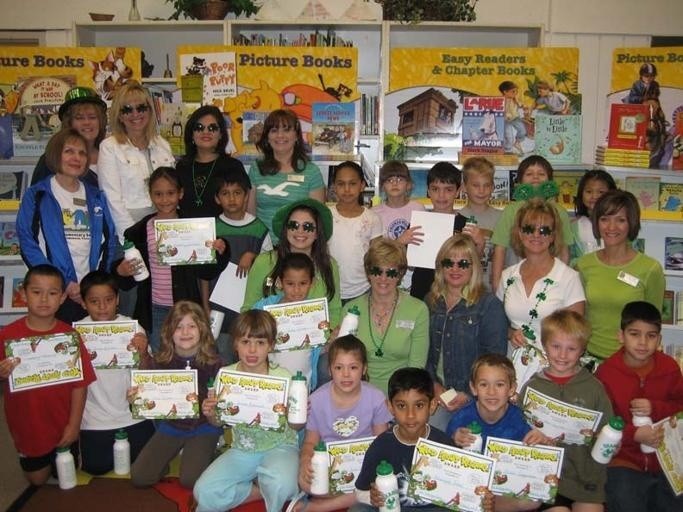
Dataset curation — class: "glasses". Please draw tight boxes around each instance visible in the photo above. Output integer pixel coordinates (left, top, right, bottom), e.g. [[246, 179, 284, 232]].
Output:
[[192, 123, 221, 132], [367, 265, 399, 279], [285, 221, 317, 233], [520, 223, 554, 238], [384, 178, 407, 184], [120, 103, 150, 115], [441, 258, 472, 270]]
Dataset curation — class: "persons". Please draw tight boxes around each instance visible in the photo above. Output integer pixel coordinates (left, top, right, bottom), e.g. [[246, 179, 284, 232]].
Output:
[[15, 81, 671, 390], [443, 350, 552, 512], [0, 261, 96, 488], [68, 269, 155, 474], [352, 363, 496, 512], [123, 300, 221, 489], [590, 299, 682, 511], [285, 334, 394, 512], [512, 307, 624, 512], [188, 307, 301, 512]]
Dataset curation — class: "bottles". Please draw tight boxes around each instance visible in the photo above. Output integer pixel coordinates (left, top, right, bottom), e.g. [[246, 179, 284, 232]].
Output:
[[337, 304, 360, 338], [523, 330, 536, 345], [462, 420, 483, 454], [374, 460, 401, 511], [128, 1, 140, 21], [206, 309, 225, 340], [286, 370, 307, 424], [54, 445, 77, 489], [631, 408, 655, 453], [590, 415, 625, 464], [206, 375, 215, 397], [309, 438, 329, 495], [462, 213, 477, 234], [121, 238, 149, 281], [112, 428, 130, 475]]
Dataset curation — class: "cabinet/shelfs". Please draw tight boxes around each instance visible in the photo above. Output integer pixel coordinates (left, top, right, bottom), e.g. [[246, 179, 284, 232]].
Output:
[[0, 156, 41, 314], [71, 21, 228, 102], [229, 18, 386, 196], [387, 21, 546, 201], [596, 164, 683, 374]]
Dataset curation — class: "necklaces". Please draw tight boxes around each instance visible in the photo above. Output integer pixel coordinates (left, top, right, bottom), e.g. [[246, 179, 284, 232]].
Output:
[[173, 353, 199, 370]]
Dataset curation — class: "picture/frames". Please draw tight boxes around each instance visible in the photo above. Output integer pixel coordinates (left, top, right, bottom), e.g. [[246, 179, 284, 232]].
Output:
[[608, 102, 650, 149], [663, 234, 683, 273], [661, 288, 678, 325]]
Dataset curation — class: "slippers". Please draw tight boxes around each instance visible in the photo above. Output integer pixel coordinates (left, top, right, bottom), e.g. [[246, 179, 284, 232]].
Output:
[[285, 491, 310, 512]]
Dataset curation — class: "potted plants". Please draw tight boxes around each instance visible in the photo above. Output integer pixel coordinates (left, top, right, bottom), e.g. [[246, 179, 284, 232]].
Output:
[[165, 0, 262, 22]]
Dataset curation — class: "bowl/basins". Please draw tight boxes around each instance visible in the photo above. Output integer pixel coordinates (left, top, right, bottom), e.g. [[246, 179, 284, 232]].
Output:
[[89, 13, 114, 22]]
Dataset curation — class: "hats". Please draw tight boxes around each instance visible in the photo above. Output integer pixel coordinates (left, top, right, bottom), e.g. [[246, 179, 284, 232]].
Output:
[[58, 87, 107, 121], [272, 199, 333, 242]]
